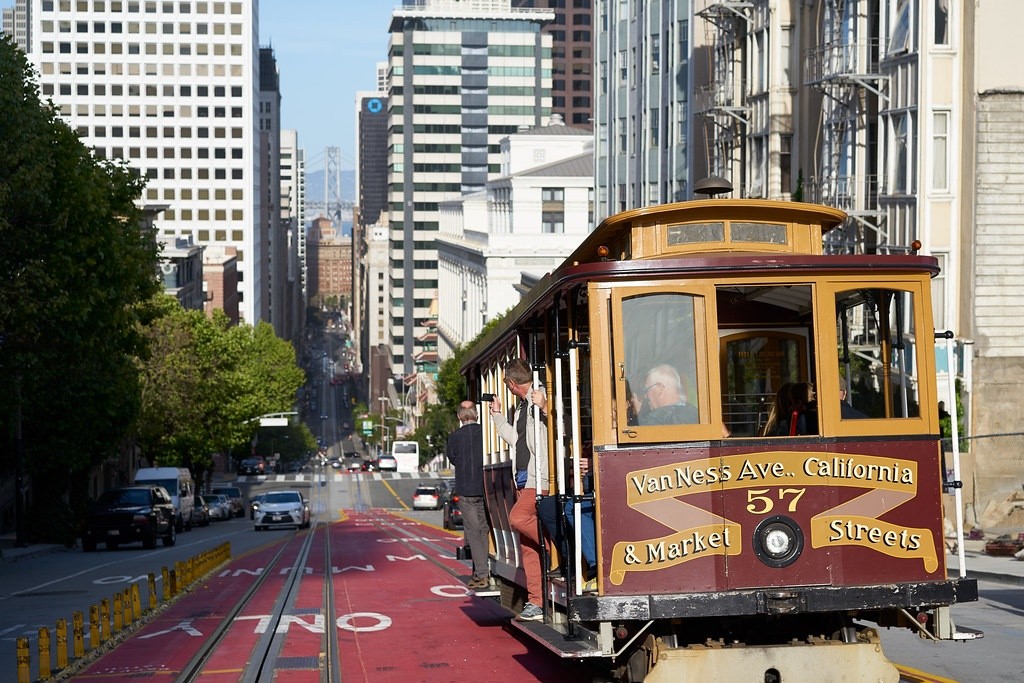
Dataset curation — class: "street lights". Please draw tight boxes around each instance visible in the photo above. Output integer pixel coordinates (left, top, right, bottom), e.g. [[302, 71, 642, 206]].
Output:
[[373, 424, 390, 454]]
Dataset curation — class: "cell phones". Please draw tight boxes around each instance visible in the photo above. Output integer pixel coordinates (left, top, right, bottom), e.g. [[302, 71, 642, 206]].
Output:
[[482, 394, 496, 401]]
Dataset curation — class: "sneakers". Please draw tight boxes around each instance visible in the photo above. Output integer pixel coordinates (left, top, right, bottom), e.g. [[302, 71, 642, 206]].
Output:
[[516, 603, 543, 621]]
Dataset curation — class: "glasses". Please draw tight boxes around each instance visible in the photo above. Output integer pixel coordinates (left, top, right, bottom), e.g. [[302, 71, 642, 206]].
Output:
[[641, 383, 666, 399], [503, 378, 513, 384]]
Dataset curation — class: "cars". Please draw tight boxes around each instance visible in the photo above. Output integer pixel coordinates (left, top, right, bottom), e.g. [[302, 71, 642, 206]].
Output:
[[192, 486, 245, 526], [248, 490, 311, 532], [359, 459, 378, 471], [377, 455, 398, 472], [238, 456, 269, 475], [412, 484, 445, 511], [346, 466, 358, 474], [322, 455, 343, 468], [442, 488, 466, 531]]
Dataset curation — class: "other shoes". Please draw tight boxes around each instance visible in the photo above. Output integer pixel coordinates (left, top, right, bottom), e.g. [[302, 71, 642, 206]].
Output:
[[546, 562, 567, 578], [466, 577, 489, 588], [587, 565, 598, 581]]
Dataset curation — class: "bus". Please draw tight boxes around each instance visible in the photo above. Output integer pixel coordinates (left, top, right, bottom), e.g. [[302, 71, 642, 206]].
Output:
[[451, 174, 976, 683], [392, 440, 420, 473]]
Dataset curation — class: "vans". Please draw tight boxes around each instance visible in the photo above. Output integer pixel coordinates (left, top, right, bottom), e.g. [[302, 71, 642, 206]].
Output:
[[134, 466, 196, 533]]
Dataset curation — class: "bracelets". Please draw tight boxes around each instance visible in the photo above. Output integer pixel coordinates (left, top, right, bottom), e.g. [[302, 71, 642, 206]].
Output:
[[493, 410, 501, 413]]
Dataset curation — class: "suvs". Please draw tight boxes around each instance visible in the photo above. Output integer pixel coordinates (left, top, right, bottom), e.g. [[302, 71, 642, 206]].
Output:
[[79, 484, 176, 550]]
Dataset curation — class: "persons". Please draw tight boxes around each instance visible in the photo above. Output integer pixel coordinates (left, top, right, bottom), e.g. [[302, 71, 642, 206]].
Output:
[[488, 359, 597, 621], [723, 372, 868, 438], [445, 401, 490, 589], [611, 364, 700, 426]]
